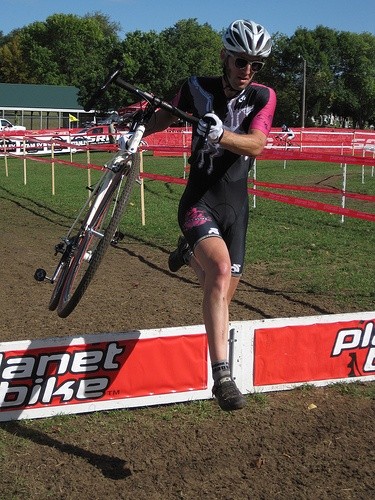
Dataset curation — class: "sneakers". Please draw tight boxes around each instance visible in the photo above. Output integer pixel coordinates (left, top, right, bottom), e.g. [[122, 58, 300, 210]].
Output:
[[168, 235, 194, 273], [212, 375, 246, 411]]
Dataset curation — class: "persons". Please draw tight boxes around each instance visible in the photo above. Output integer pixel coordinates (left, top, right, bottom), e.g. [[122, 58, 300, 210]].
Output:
[[277, 125, 295, 150], [119, 20, 278, 411]]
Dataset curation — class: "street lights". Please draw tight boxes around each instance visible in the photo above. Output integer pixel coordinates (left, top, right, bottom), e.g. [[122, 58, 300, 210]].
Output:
[[296, 51, 307, 128]]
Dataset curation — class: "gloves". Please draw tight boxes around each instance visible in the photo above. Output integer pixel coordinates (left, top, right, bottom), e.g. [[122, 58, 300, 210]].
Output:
[[197, 113, 224, 144], [118, 133, 133, 150]]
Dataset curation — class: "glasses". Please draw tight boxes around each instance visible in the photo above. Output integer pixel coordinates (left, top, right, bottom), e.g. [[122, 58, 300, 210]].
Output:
[[226, 50, 264, 73]]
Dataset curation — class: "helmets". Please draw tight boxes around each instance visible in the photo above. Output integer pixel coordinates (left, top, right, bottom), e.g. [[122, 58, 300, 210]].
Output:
[[223, 19, 272, 58]]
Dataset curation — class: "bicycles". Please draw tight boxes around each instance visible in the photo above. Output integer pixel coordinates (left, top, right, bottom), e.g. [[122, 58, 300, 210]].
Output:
[[32, 66, 210, 320], [272, 135, 298, 151]]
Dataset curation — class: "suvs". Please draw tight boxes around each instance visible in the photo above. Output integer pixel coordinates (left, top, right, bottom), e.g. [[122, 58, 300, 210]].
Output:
[[0, 118, 27, 130], [77, 125, 113, 134]]
